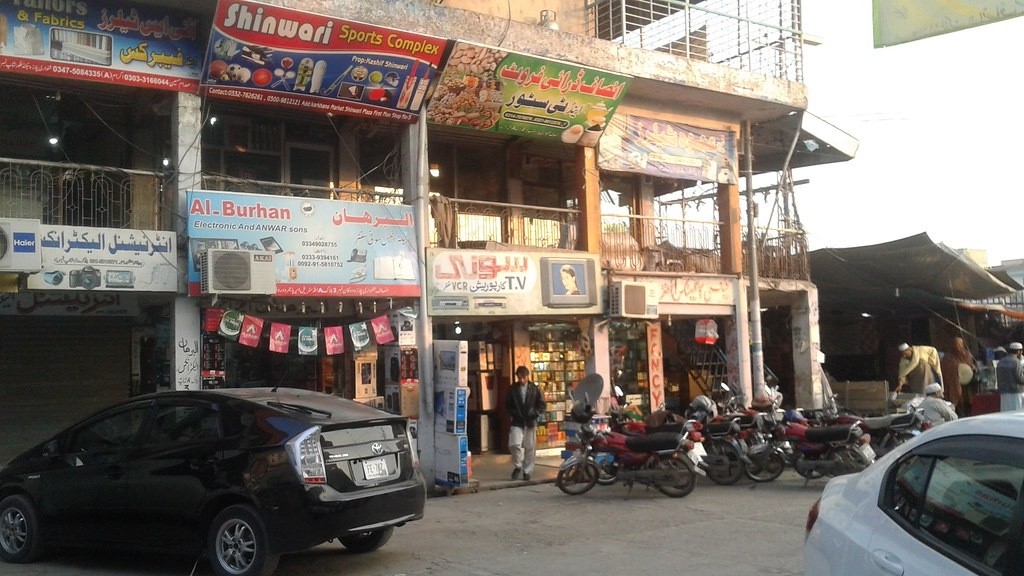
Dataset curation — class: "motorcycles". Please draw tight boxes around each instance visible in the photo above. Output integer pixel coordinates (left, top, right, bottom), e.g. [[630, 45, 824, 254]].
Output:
[[554, 382, 936, 498]]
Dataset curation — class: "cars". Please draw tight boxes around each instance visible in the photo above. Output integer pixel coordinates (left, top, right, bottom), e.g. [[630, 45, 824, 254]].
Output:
[[0, 387, 427, 576], [802, 408, 1024, 576]]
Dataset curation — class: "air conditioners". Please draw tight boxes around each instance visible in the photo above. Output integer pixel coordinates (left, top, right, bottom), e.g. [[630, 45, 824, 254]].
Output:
[[200, 249, 275, 294], [610, 281, 659, 319], [0, 218, 41, 272]]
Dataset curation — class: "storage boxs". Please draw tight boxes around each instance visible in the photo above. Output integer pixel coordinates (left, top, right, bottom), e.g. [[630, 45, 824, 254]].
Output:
[[433, 339, 470, 486]]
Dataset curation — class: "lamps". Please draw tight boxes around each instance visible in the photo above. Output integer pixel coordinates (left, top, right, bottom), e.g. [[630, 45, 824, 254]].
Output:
[[538, 10, 559, 31]]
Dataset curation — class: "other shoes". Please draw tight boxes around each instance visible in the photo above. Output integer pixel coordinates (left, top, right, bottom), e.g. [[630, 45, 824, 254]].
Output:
[[512, 468, 520, 479], [523, 474, 530, 480]]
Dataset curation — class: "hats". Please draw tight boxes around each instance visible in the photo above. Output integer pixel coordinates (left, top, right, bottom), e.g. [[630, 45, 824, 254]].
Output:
[[925, 383, 941, 393], [1009, 343, 1022, 349], [899, 343, 909, 351]]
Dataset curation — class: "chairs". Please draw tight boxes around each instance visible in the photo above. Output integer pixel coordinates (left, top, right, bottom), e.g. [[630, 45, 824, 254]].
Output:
[[651, 246, 683, 272]]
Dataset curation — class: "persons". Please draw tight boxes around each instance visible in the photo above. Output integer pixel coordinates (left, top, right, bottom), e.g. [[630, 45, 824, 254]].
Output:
[[505, 365, 548, 480], [918, 383, 959, 428], [939, 337, 973, 419], [895, 342, 944, 398], [995, 341, 1024, 410]]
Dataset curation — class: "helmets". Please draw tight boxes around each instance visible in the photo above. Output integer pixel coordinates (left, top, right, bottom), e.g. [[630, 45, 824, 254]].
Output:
[[692, 394, 711, 412]]
